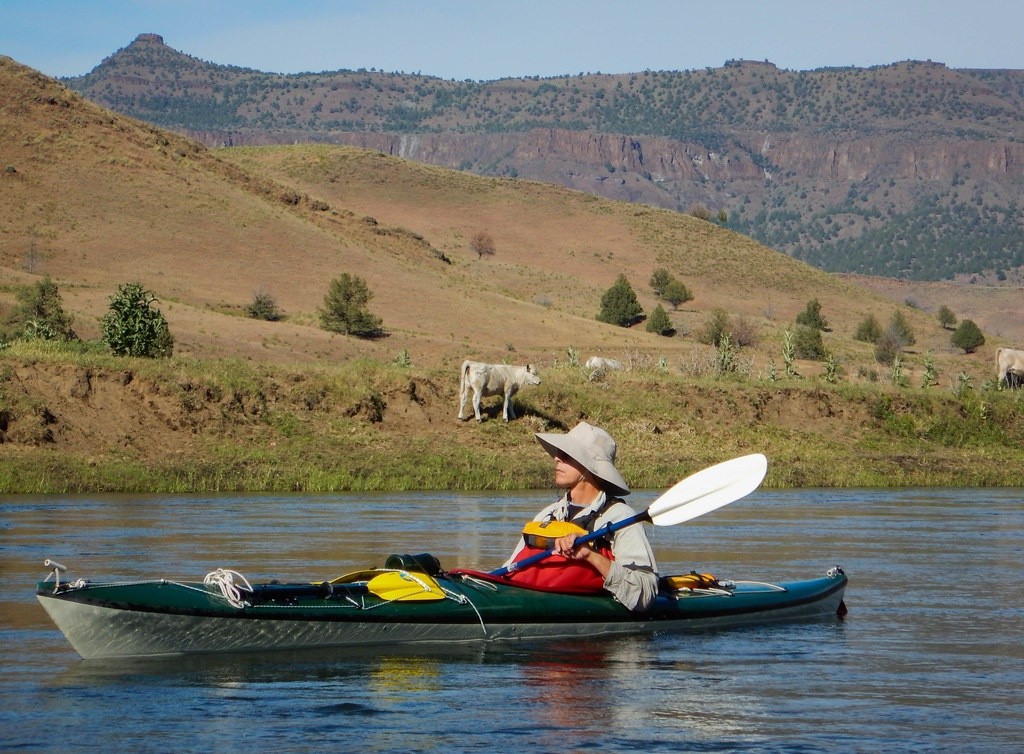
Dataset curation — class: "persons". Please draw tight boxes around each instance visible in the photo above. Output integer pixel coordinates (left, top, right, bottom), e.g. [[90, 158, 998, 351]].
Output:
[[501, 421, 659, 612]]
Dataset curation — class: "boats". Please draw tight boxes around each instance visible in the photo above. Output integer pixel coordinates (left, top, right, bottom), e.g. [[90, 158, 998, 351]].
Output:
[[35, 559, 849, 659]]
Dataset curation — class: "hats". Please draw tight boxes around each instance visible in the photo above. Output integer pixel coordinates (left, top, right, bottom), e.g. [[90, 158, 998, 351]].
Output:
[[534, 421, 630, 497]]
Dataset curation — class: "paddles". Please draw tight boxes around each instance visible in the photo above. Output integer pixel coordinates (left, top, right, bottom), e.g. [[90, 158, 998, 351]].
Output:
[[484, 450, 770, 578]]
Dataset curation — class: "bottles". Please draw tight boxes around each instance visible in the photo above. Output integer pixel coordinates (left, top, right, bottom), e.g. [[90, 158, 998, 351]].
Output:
[[385, 553, 441, 577]]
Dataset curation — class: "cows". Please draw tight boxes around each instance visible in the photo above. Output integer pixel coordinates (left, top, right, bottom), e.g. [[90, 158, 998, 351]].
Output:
[[995, 347, 1024, 392], [457, 359, 542, 424], [585, 356, 625, 371]]
